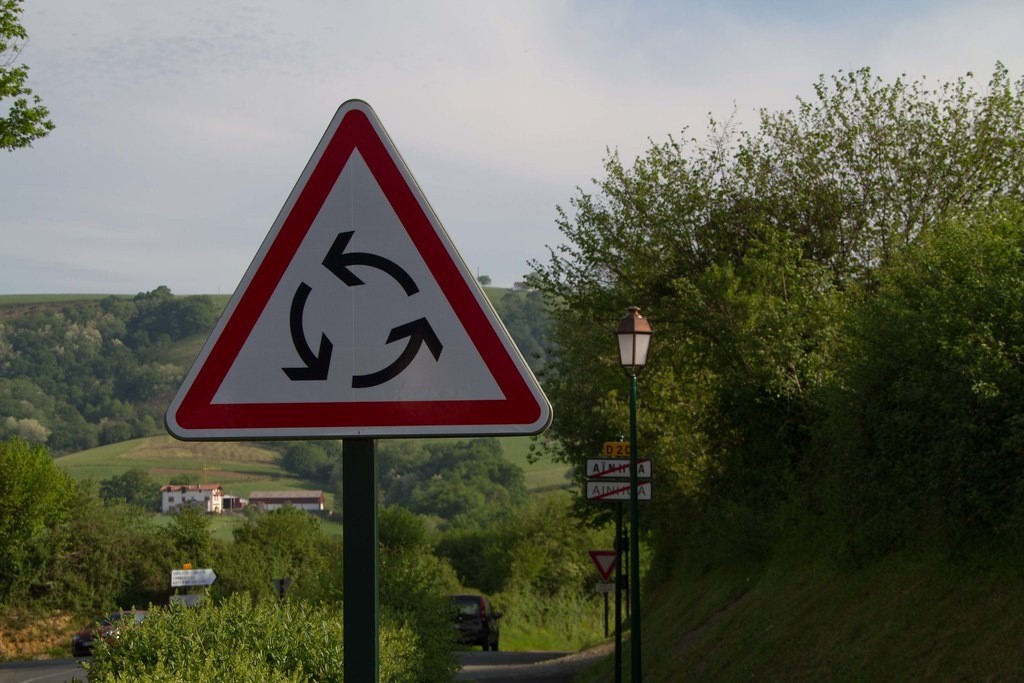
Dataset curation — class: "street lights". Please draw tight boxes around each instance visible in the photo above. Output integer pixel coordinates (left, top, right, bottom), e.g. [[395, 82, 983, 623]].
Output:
[[616, 307, 652, 682]]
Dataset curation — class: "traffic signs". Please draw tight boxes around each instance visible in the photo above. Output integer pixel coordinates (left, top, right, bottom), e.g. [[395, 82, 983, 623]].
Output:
[[171, 569, 217, 587]]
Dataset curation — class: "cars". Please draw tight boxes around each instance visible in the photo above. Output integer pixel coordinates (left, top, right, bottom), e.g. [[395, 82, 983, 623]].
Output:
[[71, 611, 147, 658]]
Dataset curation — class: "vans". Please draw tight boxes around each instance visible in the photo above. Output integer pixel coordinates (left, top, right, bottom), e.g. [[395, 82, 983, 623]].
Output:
[[444, 595, 504, 652]]
